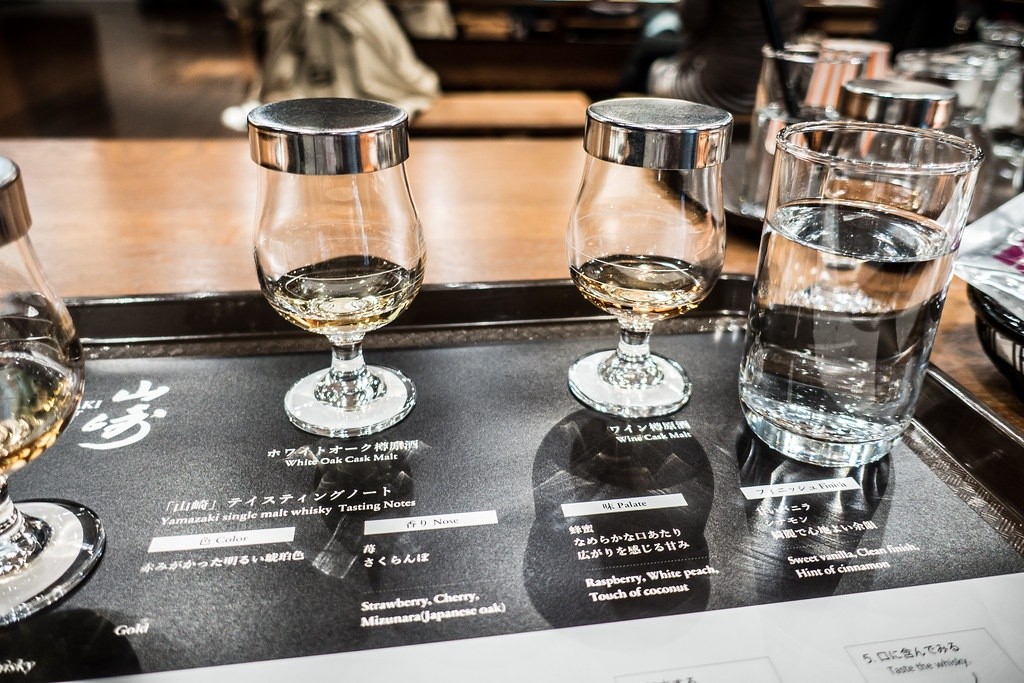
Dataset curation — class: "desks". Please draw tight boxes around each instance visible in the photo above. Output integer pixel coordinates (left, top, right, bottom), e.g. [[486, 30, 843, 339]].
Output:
[[0, 137, 1024, 439]]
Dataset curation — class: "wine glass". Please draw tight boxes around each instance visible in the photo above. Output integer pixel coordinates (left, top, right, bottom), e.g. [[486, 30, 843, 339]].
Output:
[[0, 156, 108, 629], [566, 97, 731, 416], [246, 97, 426, 438]]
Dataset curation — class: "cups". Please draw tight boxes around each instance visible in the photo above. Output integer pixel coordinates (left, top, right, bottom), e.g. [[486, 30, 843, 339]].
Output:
[[734, 20, 1023, 469]]
